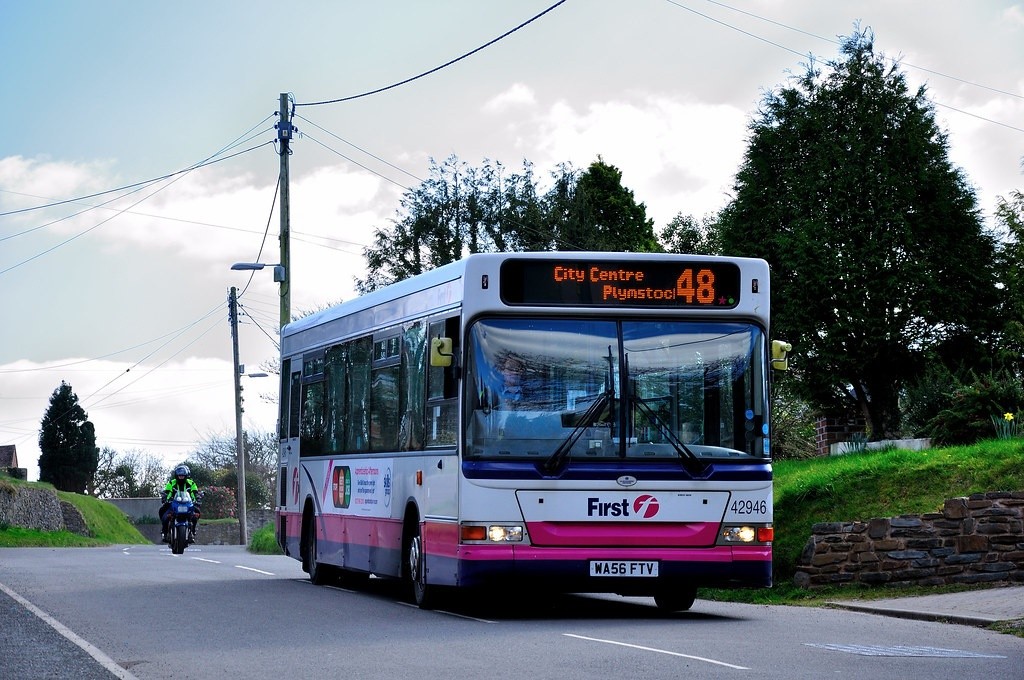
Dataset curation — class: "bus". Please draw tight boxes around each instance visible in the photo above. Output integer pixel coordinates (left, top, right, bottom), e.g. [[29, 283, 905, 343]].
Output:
[[275, 251, 794, 611]]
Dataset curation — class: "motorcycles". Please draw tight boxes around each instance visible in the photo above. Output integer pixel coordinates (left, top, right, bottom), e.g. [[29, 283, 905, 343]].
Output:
[[159, 480, 206, 554]]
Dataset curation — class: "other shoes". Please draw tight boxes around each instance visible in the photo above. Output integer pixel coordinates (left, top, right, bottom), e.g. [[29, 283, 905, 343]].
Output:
[[192, 529, 196, 535], [162, 533, 167, 540], [189, 533, 195, 542]]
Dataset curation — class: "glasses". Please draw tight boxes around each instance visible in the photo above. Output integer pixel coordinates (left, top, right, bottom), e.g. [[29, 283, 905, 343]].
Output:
[[504, 366, 521, 372]]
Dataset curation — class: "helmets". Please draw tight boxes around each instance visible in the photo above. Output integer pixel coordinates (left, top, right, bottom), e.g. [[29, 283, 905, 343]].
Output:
[[175, 466, 188, 476], [174, 464, 190, 477]]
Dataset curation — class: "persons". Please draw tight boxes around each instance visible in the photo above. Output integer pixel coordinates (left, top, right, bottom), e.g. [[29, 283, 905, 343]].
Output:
[[481, 353, 544, 411], [159, 466, 202, 542]]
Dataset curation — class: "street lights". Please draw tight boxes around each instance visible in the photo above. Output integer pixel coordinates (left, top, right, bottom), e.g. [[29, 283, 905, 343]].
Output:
[[232, 263, 292, 331], [234, 373, 268, 545]]
